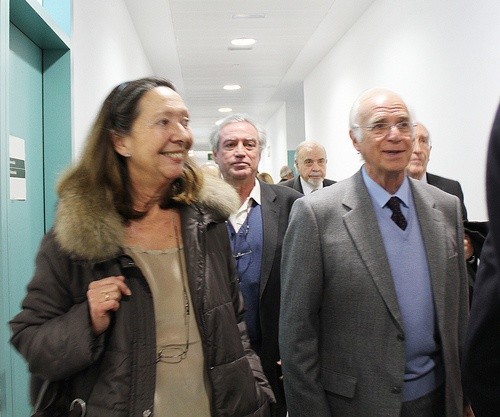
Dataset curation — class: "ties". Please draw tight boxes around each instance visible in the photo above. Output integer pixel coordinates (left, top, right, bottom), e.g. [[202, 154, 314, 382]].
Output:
[[386, 197, 407, 231]]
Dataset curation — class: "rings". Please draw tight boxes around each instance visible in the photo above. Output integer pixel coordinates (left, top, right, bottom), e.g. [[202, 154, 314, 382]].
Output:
[[104, 294, 110, 301]]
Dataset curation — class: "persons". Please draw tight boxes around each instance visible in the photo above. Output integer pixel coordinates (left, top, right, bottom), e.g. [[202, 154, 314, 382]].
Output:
[[8, 78, 276, 417], [280, 92, 466, 417], [212, 114, 303, 376], [407, 122, 483, 290], [284, 144, 338, 193], [197, 166, 294, 183]]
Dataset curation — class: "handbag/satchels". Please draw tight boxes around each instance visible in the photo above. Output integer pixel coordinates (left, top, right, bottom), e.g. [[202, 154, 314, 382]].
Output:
[[31, 378, 86, 417]]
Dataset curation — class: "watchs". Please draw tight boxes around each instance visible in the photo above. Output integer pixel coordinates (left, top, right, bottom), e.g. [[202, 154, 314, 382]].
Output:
[[467, 256, 475, 264]]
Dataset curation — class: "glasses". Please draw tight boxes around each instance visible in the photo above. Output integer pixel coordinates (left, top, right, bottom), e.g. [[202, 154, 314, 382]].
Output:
[[134, 302, 190, 369], [232, 248, 252, 258], [353, 122, 411, 134]]
